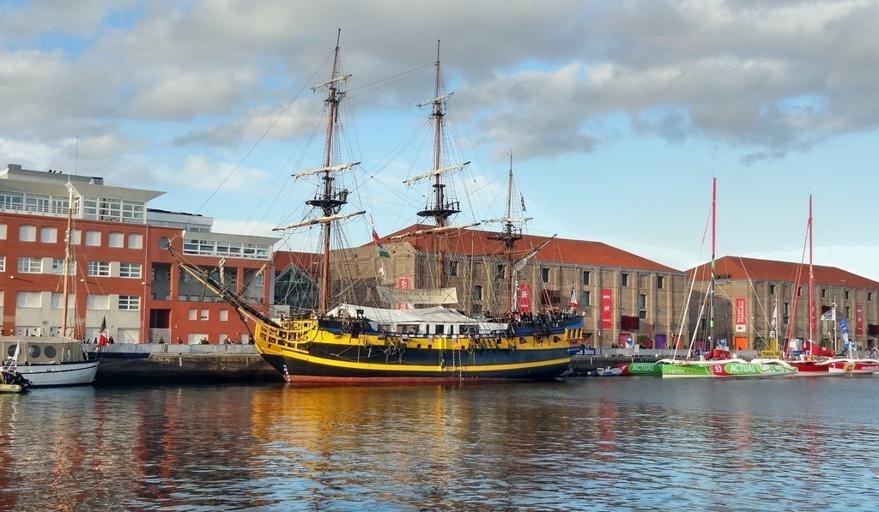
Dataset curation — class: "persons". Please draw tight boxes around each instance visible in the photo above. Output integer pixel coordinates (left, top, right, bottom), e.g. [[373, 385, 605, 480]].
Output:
[[81, 335, 114, 345], [177, 336, 182, 344], [159, 337, 165, 344], [201, 337, 209, 344], [483, 304, 577, 328], [248, 337, 255, 344], [224, 335, 233, 345]]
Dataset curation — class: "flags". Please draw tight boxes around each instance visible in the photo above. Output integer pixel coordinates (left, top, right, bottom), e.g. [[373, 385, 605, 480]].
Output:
[[99, 316, 106, 333]]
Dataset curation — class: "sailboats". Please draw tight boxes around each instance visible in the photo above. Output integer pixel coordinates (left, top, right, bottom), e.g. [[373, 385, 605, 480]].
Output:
[[786, 193, 878, 377], [602, 178, 796, 378], [165, 28, 588, 384], [0, 178, 102, 388]]
